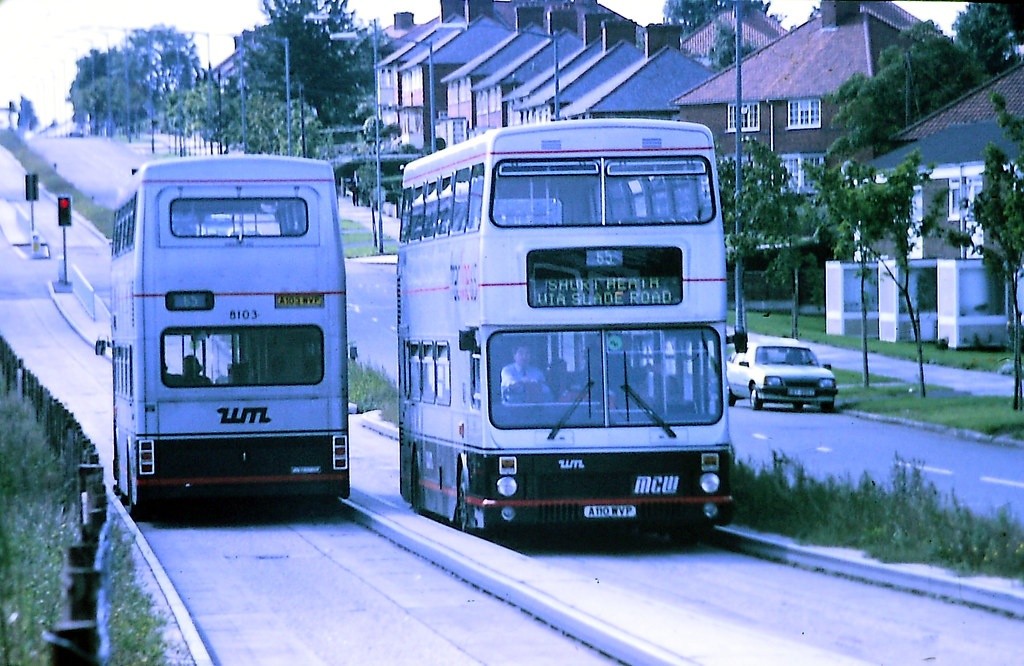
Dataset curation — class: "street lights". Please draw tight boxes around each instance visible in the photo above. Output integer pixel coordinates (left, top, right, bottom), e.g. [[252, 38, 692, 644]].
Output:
[[332, 31, 440, 157]]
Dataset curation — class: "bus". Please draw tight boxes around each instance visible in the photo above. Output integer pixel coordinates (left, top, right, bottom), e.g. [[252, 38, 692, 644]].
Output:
[[92, 155, 361, 525], [394, 117, 751, 549]]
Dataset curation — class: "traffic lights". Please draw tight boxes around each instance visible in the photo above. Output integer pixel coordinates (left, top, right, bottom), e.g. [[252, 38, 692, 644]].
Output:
[[58, 195, 74, 229]]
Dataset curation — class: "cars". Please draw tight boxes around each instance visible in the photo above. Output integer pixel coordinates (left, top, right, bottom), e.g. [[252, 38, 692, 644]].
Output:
[[726, 338, 837, 413]]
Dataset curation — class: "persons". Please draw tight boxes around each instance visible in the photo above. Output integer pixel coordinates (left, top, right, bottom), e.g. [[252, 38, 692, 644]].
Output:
[[500, 345, 554, 403], [163, 355, 212, 387]]
